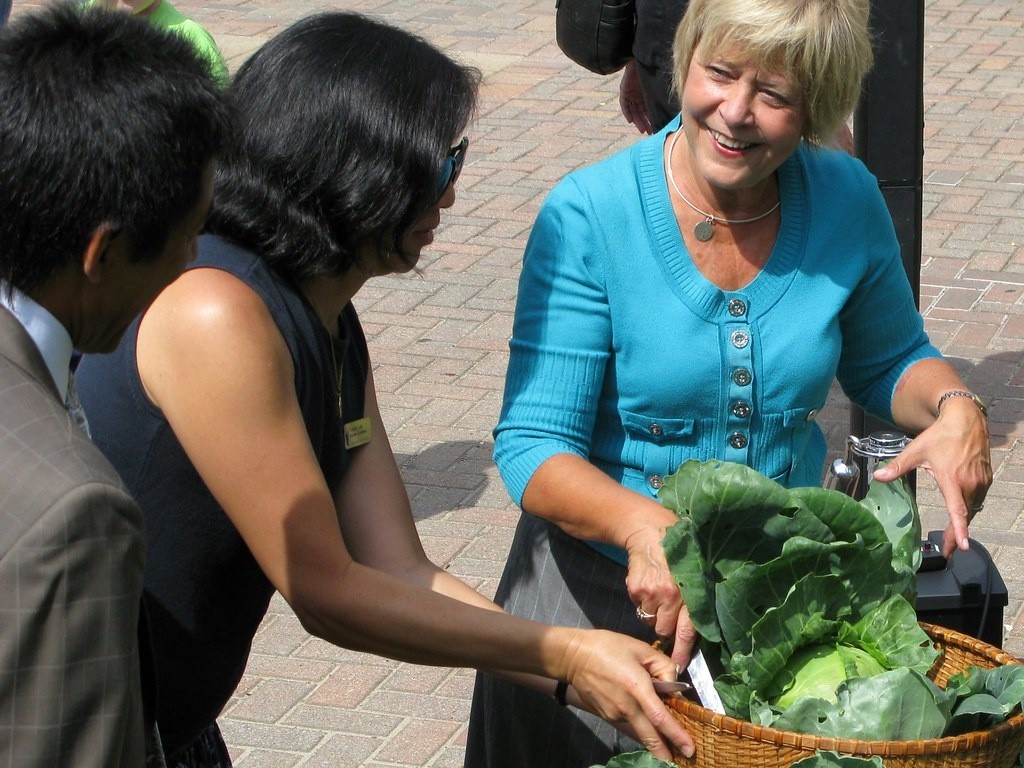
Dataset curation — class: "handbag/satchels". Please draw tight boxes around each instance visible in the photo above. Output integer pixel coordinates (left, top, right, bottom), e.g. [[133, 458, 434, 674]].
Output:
[[556, 0, 638, 76]]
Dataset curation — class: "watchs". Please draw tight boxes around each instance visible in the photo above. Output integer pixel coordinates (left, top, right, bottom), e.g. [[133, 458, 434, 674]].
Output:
[[935, 390, 990, 416]]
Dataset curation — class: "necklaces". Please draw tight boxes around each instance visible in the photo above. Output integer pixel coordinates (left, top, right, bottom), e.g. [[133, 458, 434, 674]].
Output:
[[311, 299, 343, 417], [667, 123, 781, 241]]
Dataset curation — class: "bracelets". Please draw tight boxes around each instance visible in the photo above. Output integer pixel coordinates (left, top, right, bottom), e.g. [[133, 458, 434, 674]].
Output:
[[555, 679, 569, 707]]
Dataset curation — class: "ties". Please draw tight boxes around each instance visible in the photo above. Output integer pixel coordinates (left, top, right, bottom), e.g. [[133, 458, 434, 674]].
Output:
[[63, 363, 93, 442]]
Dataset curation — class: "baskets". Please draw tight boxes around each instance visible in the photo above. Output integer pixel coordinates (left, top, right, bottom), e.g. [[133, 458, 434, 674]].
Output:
[[659, 618, 1024, 768]]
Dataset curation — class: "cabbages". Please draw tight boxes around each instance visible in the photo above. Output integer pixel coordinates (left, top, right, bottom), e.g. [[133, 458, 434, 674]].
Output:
[[589, 454, 1024, 768]]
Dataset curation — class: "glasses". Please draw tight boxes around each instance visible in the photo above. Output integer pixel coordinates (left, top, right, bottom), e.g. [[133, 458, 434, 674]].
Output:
[[431, 136, 469, 204]]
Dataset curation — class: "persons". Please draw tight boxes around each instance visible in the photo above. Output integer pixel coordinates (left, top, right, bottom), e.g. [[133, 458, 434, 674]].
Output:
[[66, 13, 695, 768], [0, 1, 231, 768], [463, 0, 992, 768]]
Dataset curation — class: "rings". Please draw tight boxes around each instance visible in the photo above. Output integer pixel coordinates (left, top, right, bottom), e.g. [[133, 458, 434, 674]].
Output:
[[635, 605, 656, 620], [972, 505, 985, 511]]
